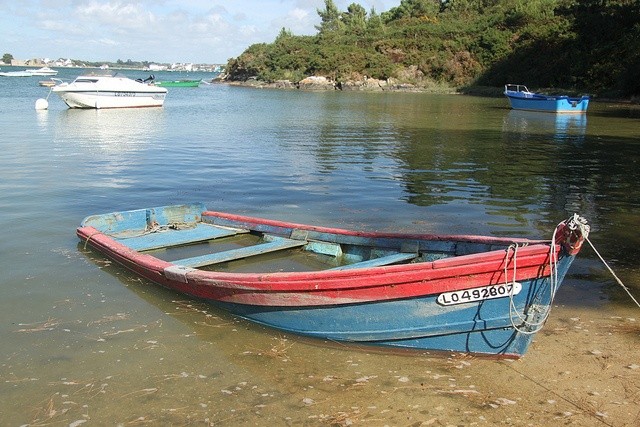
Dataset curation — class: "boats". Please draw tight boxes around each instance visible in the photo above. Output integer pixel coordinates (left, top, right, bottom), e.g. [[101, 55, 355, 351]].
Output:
[[52, 74, 168, 108], [25, 66, 58, 75], [503, 83, 590, 113], [154, 78, 203, 87], [75, 201, 589, 360], [0, 71, 32, 76]]
